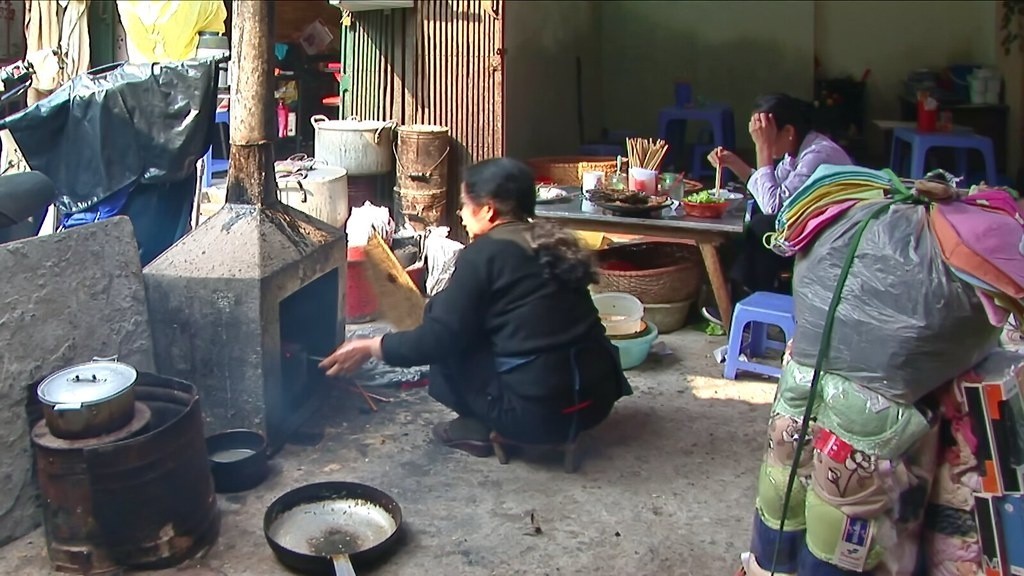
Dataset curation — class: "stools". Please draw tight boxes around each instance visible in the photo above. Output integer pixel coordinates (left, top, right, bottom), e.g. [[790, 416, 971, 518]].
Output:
[[890, 125, 998, 187], [722, 290, 796, 380], [656, 104, 732, 187], [205, 109, 229, 187]]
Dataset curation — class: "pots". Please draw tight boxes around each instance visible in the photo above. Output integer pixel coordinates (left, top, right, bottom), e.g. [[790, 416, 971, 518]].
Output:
[[37, 354, 137, 442], [263, 480, 403, 576], [311, 113, 399, 176]]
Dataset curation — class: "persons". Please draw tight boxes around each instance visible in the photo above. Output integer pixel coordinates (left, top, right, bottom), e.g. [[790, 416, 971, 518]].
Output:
[[317, 157, 634, 457], [701, 92, 855, 338]]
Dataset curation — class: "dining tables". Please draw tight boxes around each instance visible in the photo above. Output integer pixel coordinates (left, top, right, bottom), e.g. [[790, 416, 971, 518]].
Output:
[[533, 184, 746, 334]]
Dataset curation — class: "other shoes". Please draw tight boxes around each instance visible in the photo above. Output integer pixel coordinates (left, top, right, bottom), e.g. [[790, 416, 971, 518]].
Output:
[[432, 417, 490, 457], [701, 307, 751, 337]]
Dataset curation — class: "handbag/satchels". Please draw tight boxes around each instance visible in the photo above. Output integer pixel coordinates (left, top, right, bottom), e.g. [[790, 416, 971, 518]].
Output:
[[790, 193, 1003, 404]]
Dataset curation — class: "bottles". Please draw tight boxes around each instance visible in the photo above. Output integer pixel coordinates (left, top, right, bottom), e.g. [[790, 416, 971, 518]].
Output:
[[940, 105, 953, 131]]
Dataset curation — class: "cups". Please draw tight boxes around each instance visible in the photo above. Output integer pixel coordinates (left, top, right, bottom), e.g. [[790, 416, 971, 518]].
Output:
[[582, 171, 605, 196], [628, 169, 658, 196], [916, 99, 937, 132], [660, 173, 684, 200], [582, 198, 601, 213], [608, 173, 628, 190]]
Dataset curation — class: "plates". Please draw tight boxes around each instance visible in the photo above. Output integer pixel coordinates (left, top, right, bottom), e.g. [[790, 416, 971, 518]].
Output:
[[596, 201, 675, 212], [535, 187, 568, 202]]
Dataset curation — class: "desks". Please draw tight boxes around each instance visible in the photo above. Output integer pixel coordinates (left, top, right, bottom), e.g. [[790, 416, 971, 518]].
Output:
[[898, 94, 1010, 175]]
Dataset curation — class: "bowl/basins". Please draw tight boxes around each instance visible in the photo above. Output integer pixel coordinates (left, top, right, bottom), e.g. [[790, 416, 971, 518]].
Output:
[[205, 429, 268, 493], [590, 282, 699, 369], [678, 198, 729, 219], [707, 190, 744, 212]]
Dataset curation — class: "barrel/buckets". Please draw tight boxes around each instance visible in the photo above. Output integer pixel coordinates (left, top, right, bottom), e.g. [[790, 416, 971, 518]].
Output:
[[30, 373, 218, 576], [944, 63, 983, 104], [970, 66, 1003, 105], [275, 124, 448, 327]]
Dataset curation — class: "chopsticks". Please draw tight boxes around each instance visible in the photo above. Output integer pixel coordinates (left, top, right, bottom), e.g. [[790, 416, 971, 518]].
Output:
[[715, 146, 722, 199], [626, 137, 668, 171]]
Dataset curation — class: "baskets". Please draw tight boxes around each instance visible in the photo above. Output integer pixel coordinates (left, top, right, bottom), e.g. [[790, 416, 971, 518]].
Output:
[[590, 240, 707, 304]]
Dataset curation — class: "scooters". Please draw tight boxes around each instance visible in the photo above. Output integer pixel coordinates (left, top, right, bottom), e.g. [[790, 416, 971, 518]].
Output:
[[0, 44, 231, 272]]
